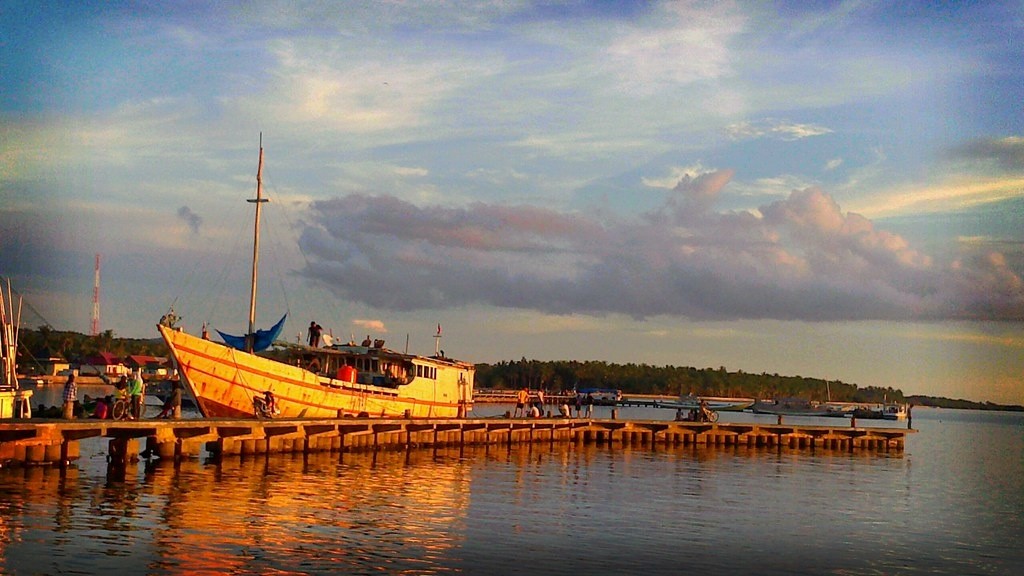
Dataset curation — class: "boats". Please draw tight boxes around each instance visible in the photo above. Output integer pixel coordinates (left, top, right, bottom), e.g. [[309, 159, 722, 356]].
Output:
[[157, 130, 476, 420], [753, 403, 880, 417], [881, 400, 908, 419], [657, 396, 754, 410]]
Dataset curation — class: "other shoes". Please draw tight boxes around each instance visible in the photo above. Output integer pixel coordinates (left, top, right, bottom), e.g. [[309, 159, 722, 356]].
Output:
[[130, 417, 139, 421]]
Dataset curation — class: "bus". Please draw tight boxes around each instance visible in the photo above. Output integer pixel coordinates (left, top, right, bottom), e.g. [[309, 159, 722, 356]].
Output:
[[576, 388, 623, 403]]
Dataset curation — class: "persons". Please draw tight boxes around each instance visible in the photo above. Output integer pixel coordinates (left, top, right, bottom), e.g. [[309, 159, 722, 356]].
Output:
[[117, 374, 141, 421], [384, 362, 395, 388], [676, 408, 683, 421], [584, 392, 594, 419], [908, 403, 915, 429], [62, 374, 84, 419], [527, 387, 544, 418], [688, 399, 708, 422], [153, 381, 182, 419], [307, 321, 324, 348], [514, 387, 529, 418], [558, 400, 572, 418], [575, 394, 582, 418]]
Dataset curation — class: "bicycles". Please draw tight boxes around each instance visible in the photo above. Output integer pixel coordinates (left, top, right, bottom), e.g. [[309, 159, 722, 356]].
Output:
[[113, 394, 145, 421]]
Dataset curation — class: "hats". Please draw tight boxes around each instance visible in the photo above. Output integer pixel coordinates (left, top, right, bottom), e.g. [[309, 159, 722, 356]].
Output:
[[524, 388, 528, 392], [539, 388, 545, 392]]
